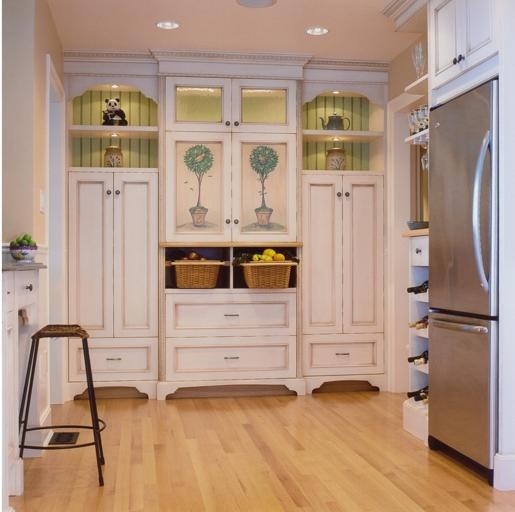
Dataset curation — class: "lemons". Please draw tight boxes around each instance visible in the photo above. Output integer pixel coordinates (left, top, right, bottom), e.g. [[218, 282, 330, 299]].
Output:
[[10, 233, 36, 246], [251, 249, 285, 261]]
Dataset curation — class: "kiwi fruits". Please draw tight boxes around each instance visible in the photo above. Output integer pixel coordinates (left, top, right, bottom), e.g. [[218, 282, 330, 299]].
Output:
[[181, 251, 208, 260]]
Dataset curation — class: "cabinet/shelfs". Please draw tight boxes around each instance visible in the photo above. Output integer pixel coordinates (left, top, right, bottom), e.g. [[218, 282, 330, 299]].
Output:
[[427, 2, 498, 109], [64, 49, 388, 402], [390, 74, 428, 439]]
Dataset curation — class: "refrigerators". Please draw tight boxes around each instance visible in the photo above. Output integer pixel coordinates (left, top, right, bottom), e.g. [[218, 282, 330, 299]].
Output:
[[429, 79, 498, 471]]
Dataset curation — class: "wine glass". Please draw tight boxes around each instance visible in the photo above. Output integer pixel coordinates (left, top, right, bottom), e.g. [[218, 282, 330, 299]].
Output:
[[10, 249, 39, 266], [408, 103, 428, 171], [409, 40, 424, 79]]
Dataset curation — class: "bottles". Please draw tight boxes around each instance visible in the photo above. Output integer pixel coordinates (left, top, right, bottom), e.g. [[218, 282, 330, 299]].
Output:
[[408, 386, 428, 401], [407, 281, 428, 294], [409, 315, 428, 329], [408, 350, 428, 366]]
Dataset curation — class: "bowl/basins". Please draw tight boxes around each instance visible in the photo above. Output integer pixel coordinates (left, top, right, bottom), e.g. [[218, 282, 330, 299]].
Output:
[[407, 220, 429, 229]]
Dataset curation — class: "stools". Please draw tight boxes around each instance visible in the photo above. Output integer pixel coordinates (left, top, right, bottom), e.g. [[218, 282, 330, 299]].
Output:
[[20, 326, 105, 487]]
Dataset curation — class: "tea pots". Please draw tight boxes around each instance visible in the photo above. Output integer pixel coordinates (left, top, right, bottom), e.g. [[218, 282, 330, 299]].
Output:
[[319, 112, 350, 130]]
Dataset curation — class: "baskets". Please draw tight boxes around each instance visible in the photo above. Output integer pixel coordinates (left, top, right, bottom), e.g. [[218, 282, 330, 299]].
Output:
[[240, 259, 298, 289], [170, 258, 225, 288]]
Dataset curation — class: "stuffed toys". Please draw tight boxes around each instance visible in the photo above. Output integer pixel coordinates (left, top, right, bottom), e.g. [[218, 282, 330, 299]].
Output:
[[103, 97, 127, 128]]
